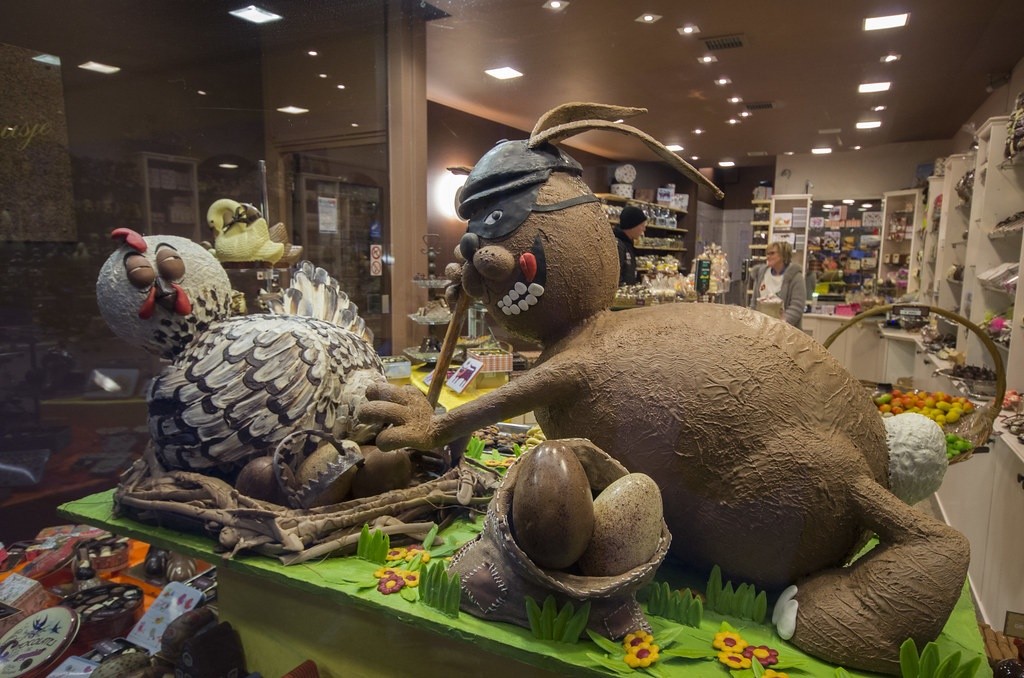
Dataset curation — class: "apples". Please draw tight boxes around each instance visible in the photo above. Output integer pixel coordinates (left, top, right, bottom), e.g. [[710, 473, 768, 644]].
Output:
[[873, 390, 973, 414]]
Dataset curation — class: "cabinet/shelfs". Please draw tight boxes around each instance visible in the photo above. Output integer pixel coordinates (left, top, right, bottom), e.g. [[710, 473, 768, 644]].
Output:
[[591, 117, 1024, 631], [295, 169, 391, 346], [133, 154, 203, 244]]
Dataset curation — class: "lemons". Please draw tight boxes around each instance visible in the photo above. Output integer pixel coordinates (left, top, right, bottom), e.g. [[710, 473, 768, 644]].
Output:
[[904, 398, 964, 429], [944, 434, 972, 460]]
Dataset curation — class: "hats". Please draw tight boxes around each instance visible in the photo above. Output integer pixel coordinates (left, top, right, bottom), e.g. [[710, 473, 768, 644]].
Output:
[[620, 206, 647, 229]]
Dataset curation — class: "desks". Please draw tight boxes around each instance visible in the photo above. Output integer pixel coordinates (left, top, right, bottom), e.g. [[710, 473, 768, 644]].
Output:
[[36, 393, 146, 433]]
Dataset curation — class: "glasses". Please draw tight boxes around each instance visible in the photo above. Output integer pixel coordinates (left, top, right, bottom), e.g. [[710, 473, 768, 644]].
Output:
[[766, 251, 774, 255]]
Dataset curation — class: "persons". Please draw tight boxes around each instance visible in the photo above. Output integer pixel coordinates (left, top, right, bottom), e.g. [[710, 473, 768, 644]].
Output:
[[750, 242, 807, 331], [613, 206, 647, 285], [805, 259, 816, 301]]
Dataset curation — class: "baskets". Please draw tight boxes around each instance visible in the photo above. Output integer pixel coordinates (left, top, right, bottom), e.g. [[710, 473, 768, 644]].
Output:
[[822, 303, 1006, 465]]
